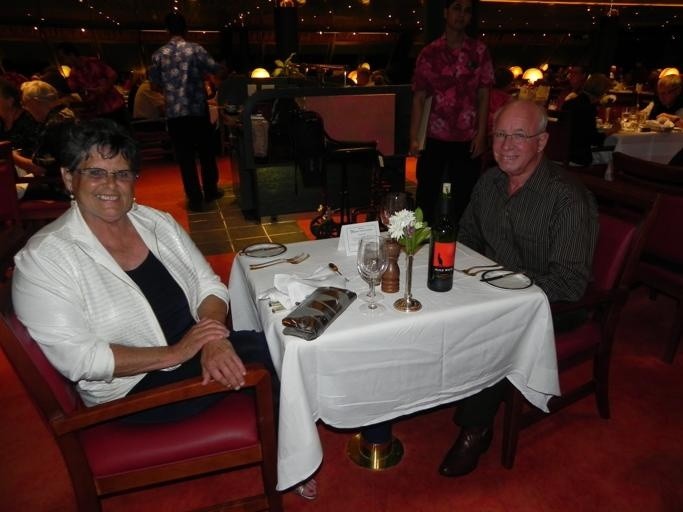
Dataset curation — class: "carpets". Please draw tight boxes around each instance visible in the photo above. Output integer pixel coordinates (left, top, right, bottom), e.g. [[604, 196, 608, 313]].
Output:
[[182, 181, 310, 256]]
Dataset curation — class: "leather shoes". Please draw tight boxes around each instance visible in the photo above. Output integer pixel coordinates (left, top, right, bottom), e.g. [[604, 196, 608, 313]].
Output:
[[439, 427, 493, 477], [189, 199, 201, 211], [205, 188, 223, 203]]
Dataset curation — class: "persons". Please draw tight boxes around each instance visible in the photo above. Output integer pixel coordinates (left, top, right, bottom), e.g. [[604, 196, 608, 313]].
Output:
[[2, 2, 224, 201], [408, 2, 497, 232], [6, 120, 317, 502], [357, 63, 386, 86], [432, 102, 603, 481], [549, 63, 682, 163]]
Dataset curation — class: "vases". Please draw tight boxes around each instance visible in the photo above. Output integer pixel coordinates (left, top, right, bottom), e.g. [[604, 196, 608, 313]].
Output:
[[394, 249, 421, 313]]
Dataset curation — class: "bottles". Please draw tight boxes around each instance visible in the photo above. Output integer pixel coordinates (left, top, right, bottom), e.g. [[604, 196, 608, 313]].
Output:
[[426, 182, 457, 292], [378, 237, 400, 294]]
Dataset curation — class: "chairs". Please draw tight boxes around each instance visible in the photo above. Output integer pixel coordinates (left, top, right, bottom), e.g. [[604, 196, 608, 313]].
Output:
[[311, 143, 381, 232], [15, 198, 71, 233], [115, 94, 168, 162], [0, 266, 283, 511], [490, 212, 638, 469], [607, 153, 682, 365], [1, 140, 33, 266]]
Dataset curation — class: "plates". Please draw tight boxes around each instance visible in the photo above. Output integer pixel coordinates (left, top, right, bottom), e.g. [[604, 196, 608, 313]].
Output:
[[482, 270, 532, 291], [242, 242, 286, 259]]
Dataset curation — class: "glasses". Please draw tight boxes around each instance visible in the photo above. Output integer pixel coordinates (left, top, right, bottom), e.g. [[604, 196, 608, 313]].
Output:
[[488, 131, 544, 144], [66, 168, 138, 183]]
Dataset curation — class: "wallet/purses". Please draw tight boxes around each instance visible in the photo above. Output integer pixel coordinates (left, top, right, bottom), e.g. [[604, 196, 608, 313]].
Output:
[[282, 287, 357, 341]]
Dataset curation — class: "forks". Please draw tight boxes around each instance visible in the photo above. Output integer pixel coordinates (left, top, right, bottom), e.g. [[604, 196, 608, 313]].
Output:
[[452, 262, 502, 274], [249, 251, 309, 271], [464, 266, 506, 278]]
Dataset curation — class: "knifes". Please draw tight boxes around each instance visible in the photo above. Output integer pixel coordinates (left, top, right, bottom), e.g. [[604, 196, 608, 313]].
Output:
[[238, 244, 283, 256], [479, 268, 526, 284]]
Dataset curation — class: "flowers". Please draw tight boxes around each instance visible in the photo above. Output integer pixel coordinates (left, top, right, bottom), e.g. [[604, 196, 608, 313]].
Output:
[[388, 209, 430, 253]]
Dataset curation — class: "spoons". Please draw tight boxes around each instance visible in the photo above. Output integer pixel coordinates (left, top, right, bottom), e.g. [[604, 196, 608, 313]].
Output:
[[326, 262, 350, 282]]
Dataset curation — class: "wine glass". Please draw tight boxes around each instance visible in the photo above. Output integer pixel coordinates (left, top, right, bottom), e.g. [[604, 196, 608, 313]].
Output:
[[356, 237, 386, 317]]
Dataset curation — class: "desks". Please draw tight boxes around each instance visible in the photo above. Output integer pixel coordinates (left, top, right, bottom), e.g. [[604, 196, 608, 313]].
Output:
[[235, 232, 562, 468]]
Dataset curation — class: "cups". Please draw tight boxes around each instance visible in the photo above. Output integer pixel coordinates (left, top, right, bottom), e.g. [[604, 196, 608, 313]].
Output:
[[619, 110, 647, 128], [379, 192, 410, 227]]
